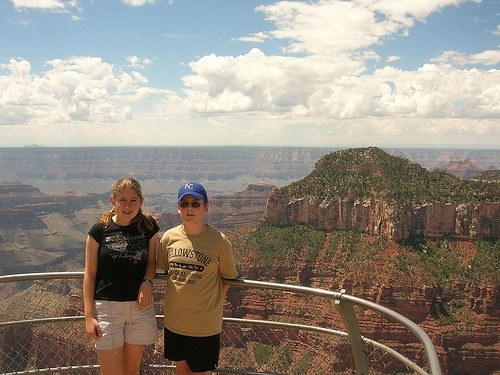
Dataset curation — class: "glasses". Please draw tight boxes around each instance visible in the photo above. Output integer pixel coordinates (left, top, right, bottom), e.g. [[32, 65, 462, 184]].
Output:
[[180, 202, 204, 208]]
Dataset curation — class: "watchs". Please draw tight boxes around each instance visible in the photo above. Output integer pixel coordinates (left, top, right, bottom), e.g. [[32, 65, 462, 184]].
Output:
[[142, 279, 153, 287]]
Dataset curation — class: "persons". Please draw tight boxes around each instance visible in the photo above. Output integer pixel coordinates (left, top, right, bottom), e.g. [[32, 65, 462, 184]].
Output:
[[156, 181, 238, 375], [83, 177, 160, 375]]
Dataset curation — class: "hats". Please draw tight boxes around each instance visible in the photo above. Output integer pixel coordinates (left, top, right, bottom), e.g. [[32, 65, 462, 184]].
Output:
[[177, 183, 207, 201]]
